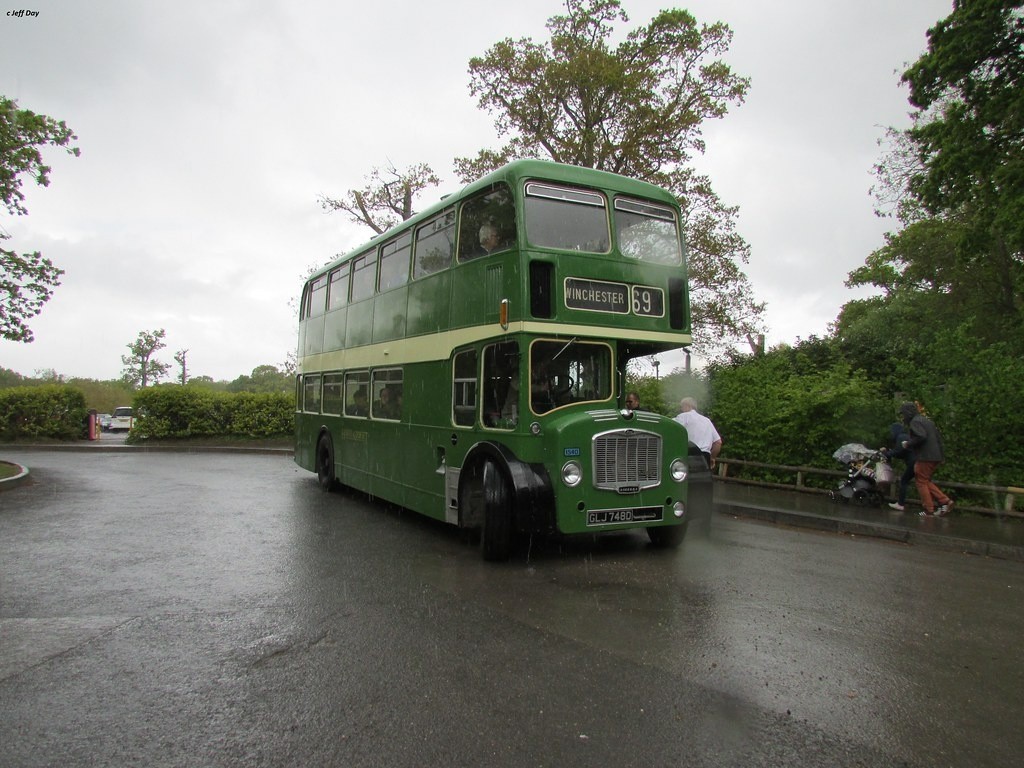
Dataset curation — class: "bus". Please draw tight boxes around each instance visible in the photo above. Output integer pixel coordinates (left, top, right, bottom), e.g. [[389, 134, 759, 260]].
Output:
[[291, 157, 693, 567]]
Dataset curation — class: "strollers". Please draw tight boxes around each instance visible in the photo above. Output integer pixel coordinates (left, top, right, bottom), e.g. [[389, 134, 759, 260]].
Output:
[[826, 443, 895, 507]]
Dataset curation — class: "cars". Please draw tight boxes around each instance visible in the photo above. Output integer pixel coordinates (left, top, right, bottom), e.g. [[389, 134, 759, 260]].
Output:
[[95, 414, 114, 430], [109, 407, 136, 430]]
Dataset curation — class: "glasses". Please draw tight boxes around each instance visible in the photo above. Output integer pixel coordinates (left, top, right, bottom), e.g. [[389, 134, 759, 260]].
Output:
[[491, 233, 503, 237]]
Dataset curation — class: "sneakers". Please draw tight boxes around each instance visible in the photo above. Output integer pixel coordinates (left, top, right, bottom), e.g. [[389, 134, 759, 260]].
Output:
[[914, 510, 937, 518], [939, 500, 954, 516]]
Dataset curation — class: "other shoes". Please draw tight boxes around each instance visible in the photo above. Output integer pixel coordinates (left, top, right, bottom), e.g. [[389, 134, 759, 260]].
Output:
[[888, 502, 905, 510], [933, 508, 941, 515]]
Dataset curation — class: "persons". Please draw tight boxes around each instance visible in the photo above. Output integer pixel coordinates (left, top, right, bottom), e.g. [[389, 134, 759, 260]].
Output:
[[901, 403, 954, 518], [471, 225, 501, 258], [880, 425, 942, 510], [348, 389, 369, 415], [669, 396, 722, 537], [374, 388, 402, 420], [624, 391, 642, 411], [852, 457, 876, 479], [502, 361, 559, 420]]
